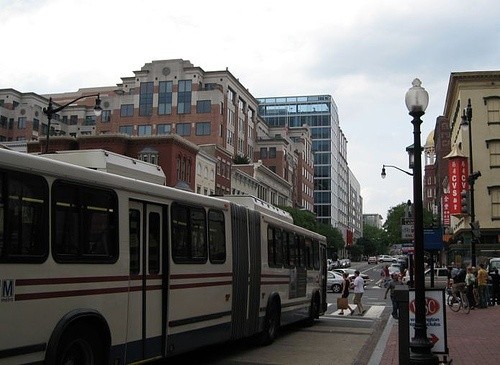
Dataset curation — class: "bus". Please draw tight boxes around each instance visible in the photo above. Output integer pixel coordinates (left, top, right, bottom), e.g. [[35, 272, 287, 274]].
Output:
[[0, 147, 329, 365]]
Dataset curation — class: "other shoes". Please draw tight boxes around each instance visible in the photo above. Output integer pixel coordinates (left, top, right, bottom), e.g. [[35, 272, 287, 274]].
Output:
[[355, 310, 366, 316], [350, 310, 354, 316], [338, 313, 344, 315], [391, 312, 399, 319]]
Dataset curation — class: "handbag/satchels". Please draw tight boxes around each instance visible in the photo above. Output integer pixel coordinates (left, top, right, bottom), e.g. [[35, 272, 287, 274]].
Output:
[[337, 298, 348, 309]]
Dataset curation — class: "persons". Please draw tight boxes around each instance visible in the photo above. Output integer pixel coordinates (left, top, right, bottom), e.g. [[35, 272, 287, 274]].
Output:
[[338, 273, 355, 315], [350, 271, 367, 316], [379, 265, 401, 319], [447, 264, 500, 310]]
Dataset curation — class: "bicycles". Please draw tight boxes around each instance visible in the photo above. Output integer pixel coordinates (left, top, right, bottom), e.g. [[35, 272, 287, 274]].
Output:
[[446, 283, 471, 314]]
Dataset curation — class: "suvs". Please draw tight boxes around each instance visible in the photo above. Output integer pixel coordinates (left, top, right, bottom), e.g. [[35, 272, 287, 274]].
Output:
[[379, 255, 398, 263]]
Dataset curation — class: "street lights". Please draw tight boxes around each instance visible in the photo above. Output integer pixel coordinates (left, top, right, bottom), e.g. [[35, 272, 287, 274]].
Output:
[[43, 92, 102, 157], [405, 77, 439, 364], [381, 143, 424, 177], [462, 100, 474, 270]]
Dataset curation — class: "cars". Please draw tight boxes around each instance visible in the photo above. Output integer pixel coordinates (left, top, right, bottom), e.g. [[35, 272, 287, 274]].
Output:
[[340, 260, 346, 268], [332, 261, 338, 268], [332, 268, 371, 289], [389, 263, 402, 275], [485, 260, 500, 277], [327, 270, 346, 294], [367, 257, 377, 264], [327, 259, 332, 269], [402, 268, 455, 290], [343, 260, 352, 268], [397, 259, 407, 265]]
